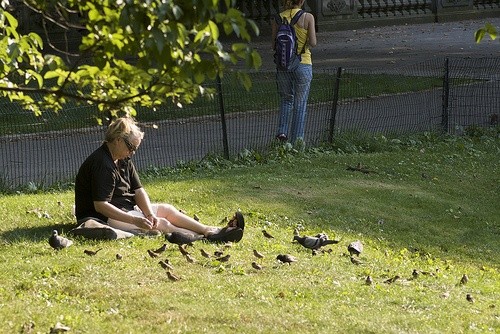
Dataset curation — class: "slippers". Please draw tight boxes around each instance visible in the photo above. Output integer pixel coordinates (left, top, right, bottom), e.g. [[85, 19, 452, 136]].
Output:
[[209, 212, 244, 242]]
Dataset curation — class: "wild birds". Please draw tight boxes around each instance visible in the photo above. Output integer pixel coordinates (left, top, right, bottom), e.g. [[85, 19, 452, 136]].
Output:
[[165, 271, 180, 282], [365, 276, 372, 286], [466, 294, 473, 302], [251, 262, 266, 271], [219, 217, 229, 226], [412, 269, 419, 278], [343, 240, 366, 267], [158, 260, 175, 270], [193, 214, 200, 223], [84, 247, 102, 258], [262, 230, 274, 239], [164, 231, 207, 250], [276, 254, 298, 266], [153, 243, 167, 255], [200, 248, 212, 258], [165, 259, 175, 269], [116, 254, 123, 260], [459, 274, 470, 286], [216, 255, 230, 263], [147, 249, 162, 259], [186, 254, 199, 264], [179, 244, 192, 256], [383, 275, 400, 284], [291, 227, 340, 257], [49, 230, 74, 251], [214, 251, 223, 258], [253, 249, 264, 259], [178, 210, 185, 214]]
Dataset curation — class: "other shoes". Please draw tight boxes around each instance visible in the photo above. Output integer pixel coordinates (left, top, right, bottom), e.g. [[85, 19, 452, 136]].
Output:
[[279, 134, 286, 140]]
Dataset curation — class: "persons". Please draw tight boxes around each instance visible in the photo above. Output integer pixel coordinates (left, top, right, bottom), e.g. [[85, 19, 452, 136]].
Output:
[[75, 118, 245, 243], [272, 0, 317, 144]]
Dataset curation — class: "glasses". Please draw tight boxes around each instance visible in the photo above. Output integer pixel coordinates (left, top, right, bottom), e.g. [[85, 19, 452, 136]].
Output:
[[123, 138, 137, 152]]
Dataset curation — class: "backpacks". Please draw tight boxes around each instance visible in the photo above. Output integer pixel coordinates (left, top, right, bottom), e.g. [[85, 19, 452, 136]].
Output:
[[273, 10, 308, 71]]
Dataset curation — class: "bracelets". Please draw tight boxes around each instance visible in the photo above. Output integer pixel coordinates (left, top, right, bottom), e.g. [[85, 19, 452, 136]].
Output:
[[147, 214, 152, 219]]
